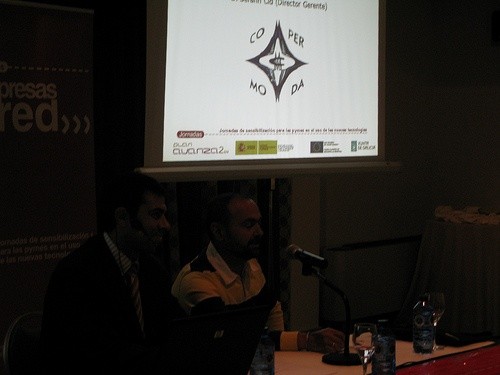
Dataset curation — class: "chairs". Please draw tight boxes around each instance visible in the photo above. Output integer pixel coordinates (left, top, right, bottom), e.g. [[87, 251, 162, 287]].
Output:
[[1, 311, 43, 369]]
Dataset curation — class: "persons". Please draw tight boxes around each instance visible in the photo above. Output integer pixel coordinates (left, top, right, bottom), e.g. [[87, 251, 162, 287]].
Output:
[[55, 172, 173, 374], [172, 191, 347, 375]]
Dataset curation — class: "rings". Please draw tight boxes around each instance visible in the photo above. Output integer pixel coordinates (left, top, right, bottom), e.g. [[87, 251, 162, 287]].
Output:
[[332, 343, 335, 347]]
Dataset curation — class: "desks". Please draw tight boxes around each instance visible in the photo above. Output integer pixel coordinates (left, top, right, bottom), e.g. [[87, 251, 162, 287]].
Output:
[[248, 339, 495, 375], [400, 219, 500, 339]]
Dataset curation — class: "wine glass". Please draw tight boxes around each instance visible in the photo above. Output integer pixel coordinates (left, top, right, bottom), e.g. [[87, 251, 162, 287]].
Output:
[[353, 323, 377, 375]]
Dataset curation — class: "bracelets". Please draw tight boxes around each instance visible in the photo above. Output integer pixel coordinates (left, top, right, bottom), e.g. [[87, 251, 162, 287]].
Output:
[[302, 330, 309, 351]]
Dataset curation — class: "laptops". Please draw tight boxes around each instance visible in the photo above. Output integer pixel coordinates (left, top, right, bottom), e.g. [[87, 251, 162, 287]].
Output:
[[160, 307, 268, 375]]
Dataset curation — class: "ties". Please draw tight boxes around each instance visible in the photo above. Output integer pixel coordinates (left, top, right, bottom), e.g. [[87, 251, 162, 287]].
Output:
[[126, 267, 147, 344]]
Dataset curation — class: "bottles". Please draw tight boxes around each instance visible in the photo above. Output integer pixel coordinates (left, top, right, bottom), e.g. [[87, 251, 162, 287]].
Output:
[[371, 320, 396, 375], [412, 293, 435, 354]]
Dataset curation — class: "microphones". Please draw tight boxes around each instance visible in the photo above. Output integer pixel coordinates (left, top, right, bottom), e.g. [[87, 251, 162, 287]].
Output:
[[142, 231, 164, 252], [285, 244, 328, 270]]
